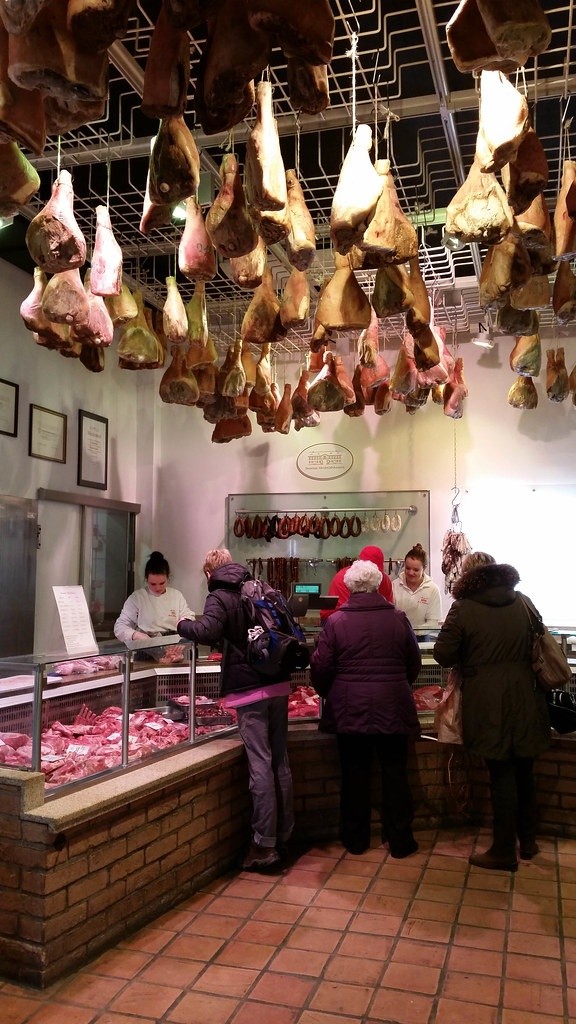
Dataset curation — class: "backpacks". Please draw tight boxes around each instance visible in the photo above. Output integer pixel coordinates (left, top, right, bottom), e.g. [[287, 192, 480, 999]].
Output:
[[216, 574, 310, 676]]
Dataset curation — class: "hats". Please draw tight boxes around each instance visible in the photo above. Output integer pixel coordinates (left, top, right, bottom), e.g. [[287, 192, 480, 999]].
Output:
[[360, 545, 384, 570]]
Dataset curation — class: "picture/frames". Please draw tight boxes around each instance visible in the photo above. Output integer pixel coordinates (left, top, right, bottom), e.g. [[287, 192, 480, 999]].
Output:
[[0, 378, 19, 438], [28, 403, 68, 464], [77, 409, 109, 490]]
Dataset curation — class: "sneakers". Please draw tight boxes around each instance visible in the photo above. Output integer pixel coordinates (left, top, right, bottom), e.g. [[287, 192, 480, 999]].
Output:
[[237, 841, 294, 869]]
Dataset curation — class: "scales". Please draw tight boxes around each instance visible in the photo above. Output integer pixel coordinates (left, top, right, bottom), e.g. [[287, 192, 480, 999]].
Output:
[[285, 582, 339, 646]]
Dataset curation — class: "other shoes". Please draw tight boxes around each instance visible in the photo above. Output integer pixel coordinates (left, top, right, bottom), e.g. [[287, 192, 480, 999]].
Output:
[[391, 841, 418, 858], [349, 848, 363, 854]]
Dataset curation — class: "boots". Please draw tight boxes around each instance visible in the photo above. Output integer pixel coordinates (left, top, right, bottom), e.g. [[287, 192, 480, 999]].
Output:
[[469, 820, 518, 871], [517, 808, 539, 860]]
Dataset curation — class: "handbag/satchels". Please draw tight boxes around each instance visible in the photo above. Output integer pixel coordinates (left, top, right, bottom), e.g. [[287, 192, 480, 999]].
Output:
[[520, 595, 573, 691], [541, 688, 575, 734]]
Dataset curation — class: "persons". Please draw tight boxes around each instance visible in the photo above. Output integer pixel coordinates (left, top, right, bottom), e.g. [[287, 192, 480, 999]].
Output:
[[309, 560, 422, 858], [176, 548, 298, 874], [390, 543, 440, 642], [432, 551, 549, 873], [113, 551, 196, 665], [319, 545, 394, 628]]
